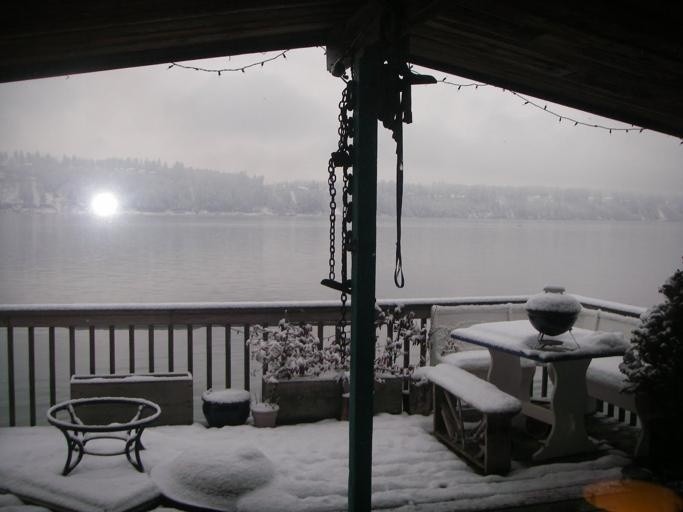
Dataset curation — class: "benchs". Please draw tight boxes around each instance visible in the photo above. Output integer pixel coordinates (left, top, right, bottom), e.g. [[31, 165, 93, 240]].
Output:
[[430, 352, 639, 473]]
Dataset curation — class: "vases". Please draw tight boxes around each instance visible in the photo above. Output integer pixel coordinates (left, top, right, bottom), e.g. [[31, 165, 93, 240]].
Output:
[[203, 390, 281, 429]]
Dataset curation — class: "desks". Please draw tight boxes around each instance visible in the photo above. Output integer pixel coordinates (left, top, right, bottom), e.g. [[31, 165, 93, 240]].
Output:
[[448, 320, 631, 462]]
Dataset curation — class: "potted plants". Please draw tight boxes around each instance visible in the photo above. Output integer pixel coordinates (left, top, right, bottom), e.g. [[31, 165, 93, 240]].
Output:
[[246, 305, 428, 426], [619, 271, 683, 459]]
